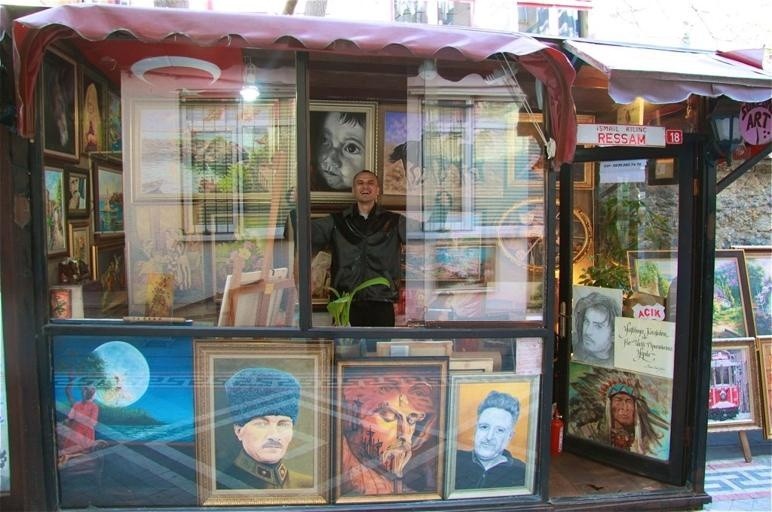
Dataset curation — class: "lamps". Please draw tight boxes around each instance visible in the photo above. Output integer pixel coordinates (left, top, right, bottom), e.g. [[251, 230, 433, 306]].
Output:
[[234, 55, 264, 104]]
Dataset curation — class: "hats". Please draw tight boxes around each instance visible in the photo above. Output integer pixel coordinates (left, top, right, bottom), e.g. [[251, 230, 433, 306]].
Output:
[[224, 368, 301, 425]]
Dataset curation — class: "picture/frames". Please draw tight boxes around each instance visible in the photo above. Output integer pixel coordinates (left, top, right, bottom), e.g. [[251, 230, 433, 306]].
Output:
[[40, 323, 199, 509], [504, 108, 599, 191], [564, 359, 673, 466], [128, 88, 507, 374], [569, 284, 618, 370], [626, 244, 762, 354], [443, 370, 541, 499], [333, 355, 451, 505], [755, 335, 772, 441], [189, 334, 335, 506], [496, 194, 594, 274], [40, 45, 128, 322], [703, 336, 762, 435], [728, 245, 771, 340]]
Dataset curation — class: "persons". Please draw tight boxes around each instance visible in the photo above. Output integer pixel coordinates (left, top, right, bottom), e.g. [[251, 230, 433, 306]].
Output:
[[67, 176, 85, 210], [311, 111, 366, 191], [454, 390, 526, 492], [342, 374, 435, 496], [287, 170, 453, 354], [62, 385, 99, 451], [574, 381, 651, 456], [217, 368, 300, 490], [573, 293, 618, 367]]
